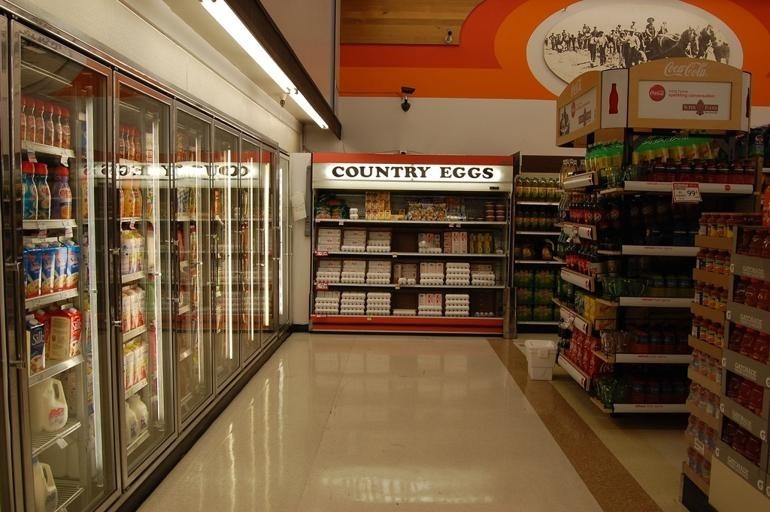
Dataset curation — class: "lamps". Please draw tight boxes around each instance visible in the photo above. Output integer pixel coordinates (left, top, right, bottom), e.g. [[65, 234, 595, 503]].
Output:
[[445, 31, 452, 43], [401, 96, 411, 113]]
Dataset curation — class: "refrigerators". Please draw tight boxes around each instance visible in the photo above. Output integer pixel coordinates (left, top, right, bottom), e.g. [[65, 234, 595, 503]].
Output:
[[0, 0, 294, 508]]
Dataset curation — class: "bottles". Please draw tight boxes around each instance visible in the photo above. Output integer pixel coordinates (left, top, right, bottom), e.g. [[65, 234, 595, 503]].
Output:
[[117, 124, 144, 274], [19, 90, 73, 221], [726, 126, 769, 467], [515, 174, 560, 231], [556, 128, 715, 408]]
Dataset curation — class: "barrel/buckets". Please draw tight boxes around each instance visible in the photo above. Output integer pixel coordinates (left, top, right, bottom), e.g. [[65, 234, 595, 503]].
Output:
[[128, 394, 148, 435], [31, 457, 59, 512], [33, 378, 68, 433], [124, 402, 139, 447]]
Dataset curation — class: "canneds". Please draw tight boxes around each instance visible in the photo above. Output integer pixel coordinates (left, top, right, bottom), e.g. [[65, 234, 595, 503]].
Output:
[[686, 211, 762, 485]]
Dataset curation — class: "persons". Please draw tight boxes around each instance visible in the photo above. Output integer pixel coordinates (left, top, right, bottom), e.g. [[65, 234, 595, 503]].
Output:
[[545, 16, 669, 70]]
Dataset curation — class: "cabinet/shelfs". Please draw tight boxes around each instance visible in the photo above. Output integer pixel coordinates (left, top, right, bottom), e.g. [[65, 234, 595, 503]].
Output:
[[0, 0, 290, 512], [308, 153, 515, 338], [677, 213, 768, 512], [511, 149, 586, 340], [555, 149, 767, 426]]
